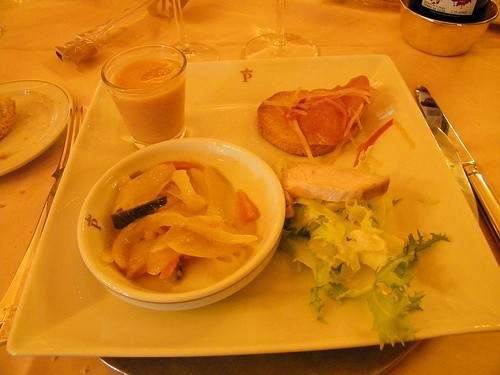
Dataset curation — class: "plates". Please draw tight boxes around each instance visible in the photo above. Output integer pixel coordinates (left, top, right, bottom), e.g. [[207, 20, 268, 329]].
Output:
[[76, 137, 286, 312], [0, 79, 73, 175], [6, 54, 500, 357]]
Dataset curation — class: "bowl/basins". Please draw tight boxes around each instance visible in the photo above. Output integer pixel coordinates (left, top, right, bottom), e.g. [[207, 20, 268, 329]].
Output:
[[400, 0, 500, 57]]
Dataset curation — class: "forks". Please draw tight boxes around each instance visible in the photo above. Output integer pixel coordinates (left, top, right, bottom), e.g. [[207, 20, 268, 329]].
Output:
[[1, 107, 83, 343]]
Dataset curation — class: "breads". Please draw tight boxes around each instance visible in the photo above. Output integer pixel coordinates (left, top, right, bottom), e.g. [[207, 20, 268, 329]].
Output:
[[256, 74, 369, 157]]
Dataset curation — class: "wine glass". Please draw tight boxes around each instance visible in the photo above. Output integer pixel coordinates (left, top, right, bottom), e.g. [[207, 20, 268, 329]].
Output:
[[243, 0, 318, 59], [166, 0, 220, 61]]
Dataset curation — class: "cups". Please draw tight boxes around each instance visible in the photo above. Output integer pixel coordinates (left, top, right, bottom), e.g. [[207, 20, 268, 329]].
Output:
[[101, 44, 187, 149]]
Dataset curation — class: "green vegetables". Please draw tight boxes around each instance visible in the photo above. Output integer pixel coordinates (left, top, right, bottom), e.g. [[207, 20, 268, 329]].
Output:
[[278, 196, 450, 348]]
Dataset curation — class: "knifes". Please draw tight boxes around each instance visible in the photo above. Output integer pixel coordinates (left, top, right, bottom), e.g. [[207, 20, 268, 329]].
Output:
[[415, 84, 500, 242]]
